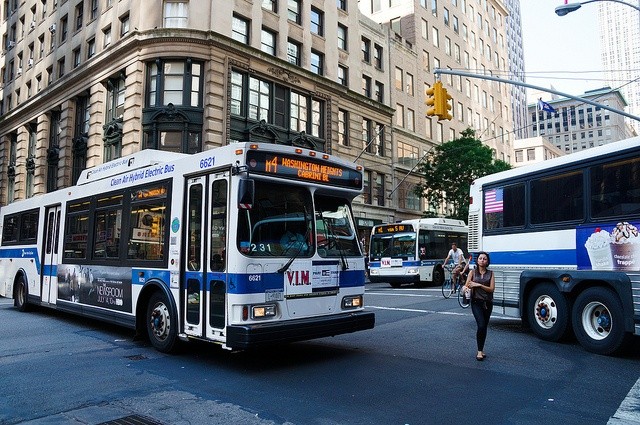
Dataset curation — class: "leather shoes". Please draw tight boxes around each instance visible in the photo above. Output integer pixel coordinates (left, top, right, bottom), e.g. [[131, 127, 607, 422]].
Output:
[[477, 350, 486, 361]]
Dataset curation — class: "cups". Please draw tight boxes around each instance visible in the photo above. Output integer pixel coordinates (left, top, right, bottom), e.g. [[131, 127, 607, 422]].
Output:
[[585, 243, 613, 271], [609, 241, 640, 272]]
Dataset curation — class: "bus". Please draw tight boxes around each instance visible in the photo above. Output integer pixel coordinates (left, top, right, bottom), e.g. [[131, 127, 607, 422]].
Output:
[[467, 135, 640, 354], [0, 141, 375, 353], [367, 218, 467, 285]]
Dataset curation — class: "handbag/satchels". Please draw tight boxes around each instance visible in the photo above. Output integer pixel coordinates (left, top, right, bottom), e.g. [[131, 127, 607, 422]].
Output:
[[460, 286, 471, 304]]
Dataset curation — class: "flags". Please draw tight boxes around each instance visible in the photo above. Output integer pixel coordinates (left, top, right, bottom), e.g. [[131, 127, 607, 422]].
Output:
[[538, 98, 557, 112]]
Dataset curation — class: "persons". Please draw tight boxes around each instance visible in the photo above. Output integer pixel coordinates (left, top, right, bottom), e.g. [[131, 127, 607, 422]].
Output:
[[364, 254, 370, 279], [465, 251, 494, 361], [442, 243, 467, 294], [460, 257, 469, 275], [280, 221, 308, 256]]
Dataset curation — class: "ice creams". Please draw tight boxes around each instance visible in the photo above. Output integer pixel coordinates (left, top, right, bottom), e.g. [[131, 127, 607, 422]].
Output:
[[610, 222, 640, 271], [584, 227, 614, 270]]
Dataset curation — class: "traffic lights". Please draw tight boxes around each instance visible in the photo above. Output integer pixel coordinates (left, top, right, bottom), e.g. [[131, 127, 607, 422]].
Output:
[[438, 88, 452, 120], [425, 79, 443, 116]]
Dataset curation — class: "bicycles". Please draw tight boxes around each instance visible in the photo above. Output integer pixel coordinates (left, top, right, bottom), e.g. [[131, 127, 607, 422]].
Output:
[[442, 264, 454, 298], [458, 284, 471, 308]]
